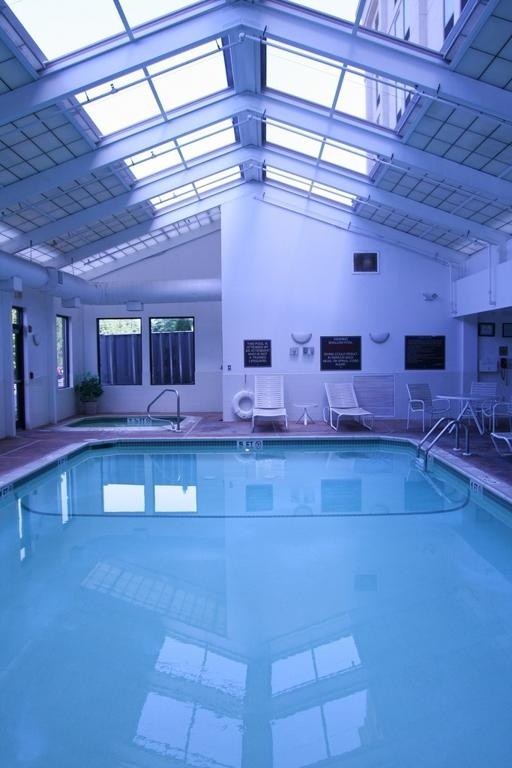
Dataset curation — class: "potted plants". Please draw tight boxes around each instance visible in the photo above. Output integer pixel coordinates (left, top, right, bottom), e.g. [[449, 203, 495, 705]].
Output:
[[74, 371, 105, 415]]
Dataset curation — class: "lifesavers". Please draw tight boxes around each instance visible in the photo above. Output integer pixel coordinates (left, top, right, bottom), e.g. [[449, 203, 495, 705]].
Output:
[[232, 390, 255, 419]]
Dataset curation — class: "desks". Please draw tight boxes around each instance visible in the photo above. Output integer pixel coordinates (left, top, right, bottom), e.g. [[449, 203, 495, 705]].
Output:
[[293, 403, 318, 426], [435, 394, 499, 435]]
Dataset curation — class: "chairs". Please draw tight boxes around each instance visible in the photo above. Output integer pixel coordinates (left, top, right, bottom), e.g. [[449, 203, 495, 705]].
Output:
[[250, 374, 288, 433], [459, 382, 497, 431], [405, 383, 450, 433], [322, 382, 374, 432]]
